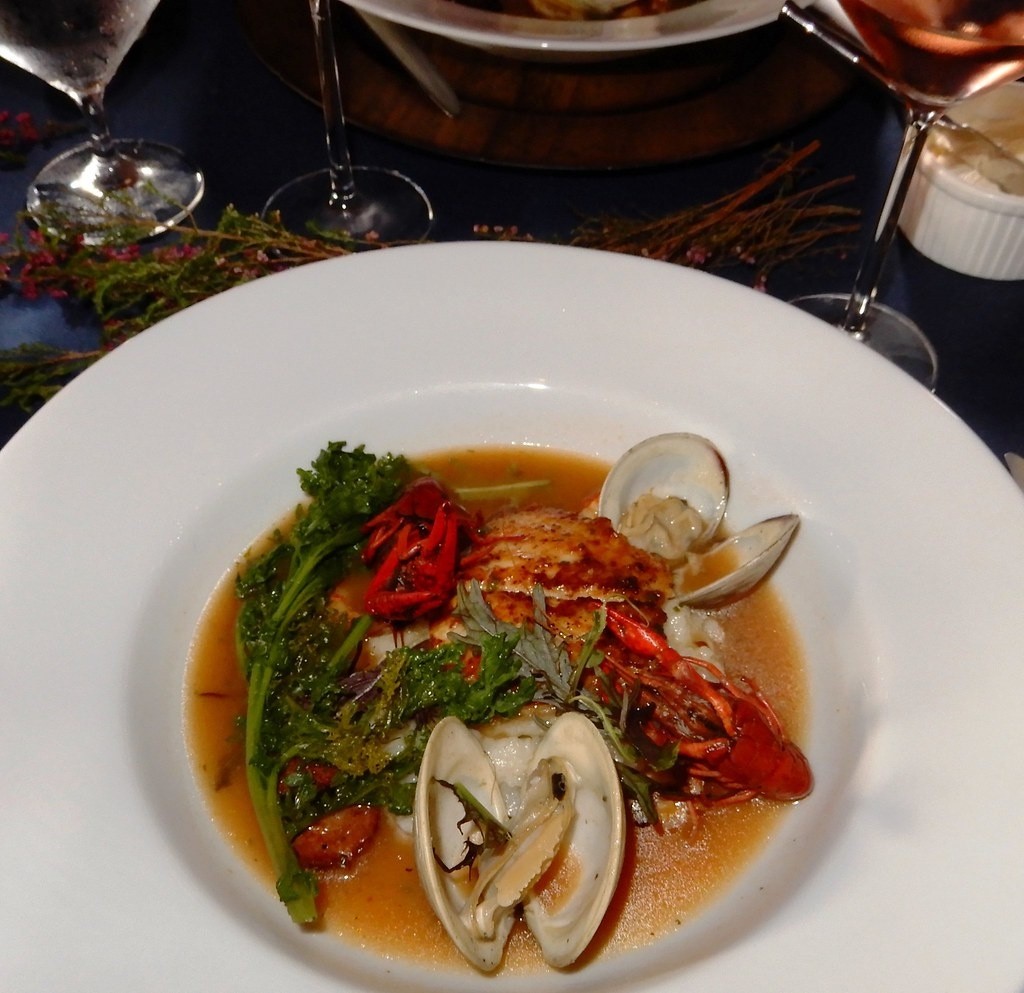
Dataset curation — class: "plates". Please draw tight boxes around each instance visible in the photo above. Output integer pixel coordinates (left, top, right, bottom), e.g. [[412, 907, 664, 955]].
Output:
[[339, 0, 789, 61], [0, 241, 1024, 993]]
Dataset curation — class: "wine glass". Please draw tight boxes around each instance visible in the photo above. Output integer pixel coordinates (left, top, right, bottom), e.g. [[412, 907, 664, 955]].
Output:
[[0, 0, 205, 247], [781, 0, 1024, 396], [261, 0, 434, 266]]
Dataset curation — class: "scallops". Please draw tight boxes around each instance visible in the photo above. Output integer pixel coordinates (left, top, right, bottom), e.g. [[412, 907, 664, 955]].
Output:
[[414, 711, 628, 970], [598, 432, 798, 602]]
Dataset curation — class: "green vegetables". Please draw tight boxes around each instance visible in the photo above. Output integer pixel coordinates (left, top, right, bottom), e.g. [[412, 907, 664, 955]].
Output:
[[227, 441, 680, 927]]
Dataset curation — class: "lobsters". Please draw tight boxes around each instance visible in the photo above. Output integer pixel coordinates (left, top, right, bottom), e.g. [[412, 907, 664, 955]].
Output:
[[362, 473, 481, 624], [602, 613, 812, 806]]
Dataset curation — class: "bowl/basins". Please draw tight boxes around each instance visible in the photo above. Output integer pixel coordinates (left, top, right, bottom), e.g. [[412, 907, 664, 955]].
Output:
[[897, 81, 1024, 281]]
